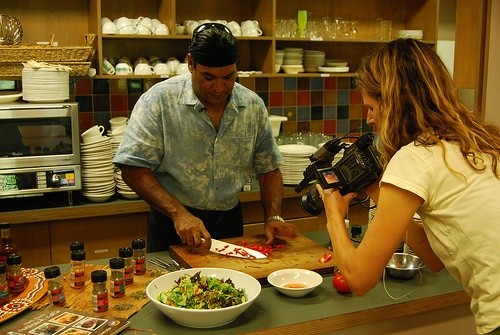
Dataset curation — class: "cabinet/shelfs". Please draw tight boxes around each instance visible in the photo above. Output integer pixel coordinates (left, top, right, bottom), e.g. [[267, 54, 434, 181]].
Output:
[[0, 212, 149, 267], [86, 0, 441, 78]]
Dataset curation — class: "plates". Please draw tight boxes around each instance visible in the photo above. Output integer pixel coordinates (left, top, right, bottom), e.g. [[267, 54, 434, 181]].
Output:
[[80, 137, 115, 202], [0, 94, 23, 104], [317, 66, 349, 72], [109, 139, 141, 199], [278, 144, 318, 184], [22, 68, 70, 103]]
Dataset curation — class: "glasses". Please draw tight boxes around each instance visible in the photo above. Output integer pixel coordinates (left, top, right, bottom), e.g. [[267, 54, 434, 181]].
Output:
[[191, 23, 232, 43]]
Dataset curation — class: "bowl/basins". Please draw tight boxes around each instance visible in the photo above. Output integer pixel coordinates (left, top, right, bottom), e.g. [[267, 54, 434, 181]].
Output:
[[385, 253, 424, 279], [275, 48, 347, 74], [267, 269, 323, 297], [147, 268, 261, 328]]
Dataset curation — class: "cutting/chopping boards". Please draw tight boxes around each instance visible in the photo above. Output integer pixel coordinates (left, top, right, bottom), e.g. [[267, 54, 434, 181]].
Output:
[[169, 233, 336, 285]]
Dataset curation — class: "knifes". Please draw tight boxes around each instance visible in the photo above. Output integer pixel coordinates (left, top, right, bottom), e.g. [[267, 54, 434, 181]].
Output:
[[192, 236, 267, 259]]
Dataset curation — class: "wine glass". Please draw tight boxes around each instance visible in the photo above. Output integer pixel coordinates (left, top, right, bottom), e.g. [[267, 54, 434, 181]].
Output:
[[274, 17, 359, 41]]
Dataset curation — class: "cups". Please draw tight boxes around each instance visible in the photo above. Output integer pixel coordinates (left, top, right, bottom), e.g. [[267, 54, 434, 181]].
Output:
[[176, 19, 263, 37], [107, 117, 129, 140], [103, 56, 190, 75], [102, 16, 169, 35], [81, 125, 107, 143], [380, 20, 392, 41]]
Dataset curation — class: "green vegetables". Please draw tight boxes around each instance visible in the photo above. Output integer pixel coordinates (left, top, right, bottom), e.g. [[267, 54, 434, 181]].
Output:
[[158, 271, 246, 310]]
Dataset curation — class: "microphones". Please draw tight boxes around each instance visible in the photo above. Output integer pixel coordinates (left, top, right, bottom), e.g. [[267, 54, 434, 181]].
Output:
[[198, 108, 207, 113]]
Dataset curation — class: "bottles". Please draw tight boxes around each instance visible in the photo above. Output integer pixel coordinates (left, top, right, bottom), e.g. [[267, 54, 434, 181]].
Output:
[[44, 265, 66, 307], [118, 246, 134, 285], [132, 238, 148, 275], [91, 270, 108, 313], [0, 222, 24, 304], [110, 258, 126, 298], [70, 241, 85, 290]]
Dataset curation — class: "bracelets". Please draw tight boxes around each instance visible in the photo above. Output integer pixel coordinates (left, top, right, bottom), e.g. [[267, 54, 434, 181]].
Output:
[[265, 216, 285, 223]]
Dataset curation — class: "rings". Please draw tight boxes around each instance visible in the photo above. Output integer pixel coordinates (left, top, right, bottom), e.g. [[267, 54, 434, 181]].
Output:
[[319, 192, 323, 198]]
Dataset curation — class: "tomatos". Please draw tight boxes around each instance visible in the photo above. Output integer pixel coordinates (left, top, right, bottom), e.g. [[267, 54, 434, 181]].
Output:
[[217, 240, 287, 259], [333, 268, 352, 294]]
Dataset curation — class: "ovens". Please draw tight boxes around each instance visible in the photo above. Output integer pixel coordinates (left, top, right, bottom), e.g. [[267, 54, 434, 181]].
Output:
[[0, 102, 82, 206]]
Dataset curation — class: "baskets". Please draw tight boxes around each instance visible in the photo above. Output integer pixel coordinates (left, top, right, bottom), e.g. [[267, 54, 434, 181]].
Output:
[[0, 31, 97, 76]]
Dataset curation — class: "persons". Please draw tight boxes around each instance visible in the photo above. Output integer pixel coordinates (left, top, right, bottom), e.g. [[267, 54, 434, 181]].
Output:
[[314, 37, 500, 334], [111, 22, 298, 254]]
